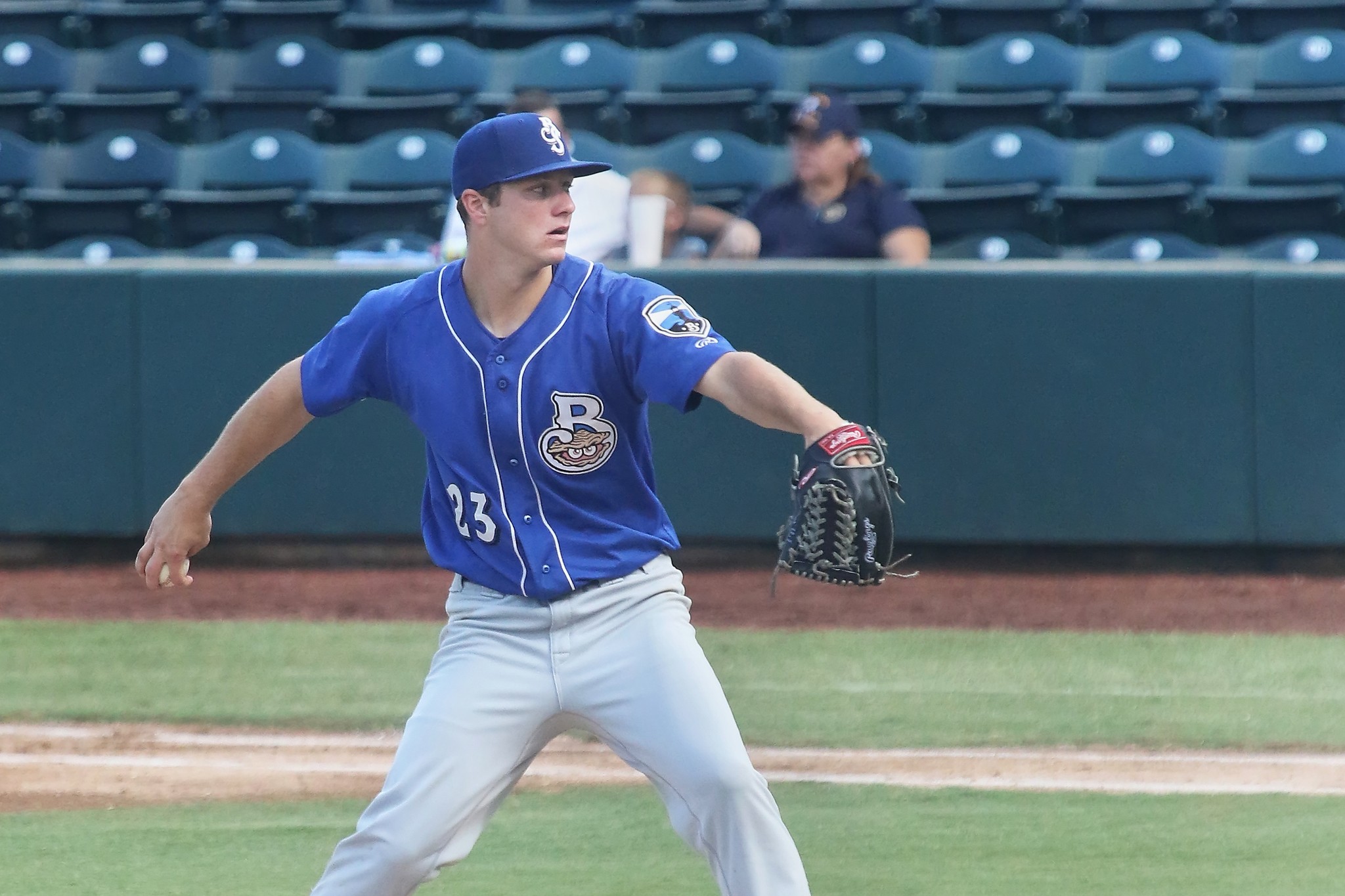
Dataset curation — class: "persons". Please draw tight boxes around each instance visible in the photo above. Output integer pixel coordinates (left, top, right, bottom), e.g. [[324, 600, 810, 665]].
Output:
[[594, 170, 706, 266], [133, 109, 922, 896], [437, 92, 763, 266], [739, 83, 932, 261]]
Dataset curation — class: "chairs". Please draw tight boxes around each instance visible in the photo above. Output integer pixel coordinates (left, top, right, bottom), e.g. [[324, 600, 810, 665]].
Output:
[[0, 0, 1345, 268]]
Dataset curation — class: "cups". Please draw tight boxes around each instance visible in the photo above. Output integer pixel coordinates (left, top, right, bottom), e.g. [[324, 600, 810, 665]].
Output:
[[627, 196, 668, 270]]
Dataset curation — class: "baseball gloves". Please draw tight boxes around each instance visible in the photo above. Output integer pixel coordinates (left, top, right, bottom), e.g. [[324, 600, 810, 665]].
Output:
[[770, 422, 921, 602]]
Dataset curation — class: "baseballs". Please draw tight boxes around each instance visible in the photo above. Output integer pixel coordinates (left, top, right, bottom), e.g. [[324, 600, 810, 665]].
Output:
[[156, 555, 190, 589]]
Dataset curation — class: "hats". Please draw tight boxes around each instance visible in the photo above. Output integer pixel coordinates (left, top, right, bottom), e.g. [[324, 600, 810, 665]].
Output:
[[785, 87, 863, 137], [451, 110, 613, 201]]
